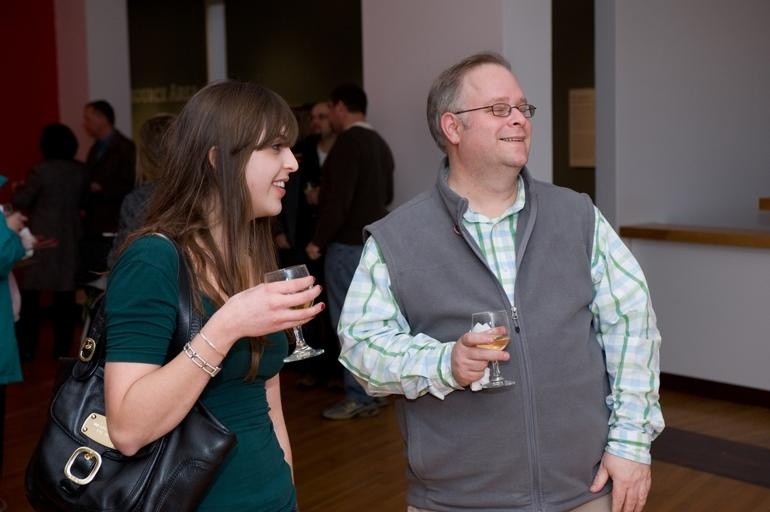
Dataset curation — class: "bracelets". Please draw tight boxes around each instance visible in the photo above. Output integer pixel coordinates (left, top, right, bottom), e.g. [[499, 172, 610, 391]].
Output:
[[198, 332, 226, 359], [183, 342, 222, 377]]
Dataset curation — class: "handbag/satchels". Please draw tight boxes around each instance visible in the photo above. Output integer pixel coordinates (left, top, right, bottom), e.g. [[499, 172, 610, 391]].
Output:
[[23, 363, 236, 512]]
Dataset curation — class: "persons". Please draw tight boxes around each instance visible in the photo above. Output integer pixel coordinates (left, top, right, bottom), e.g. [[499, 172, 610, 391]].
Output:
[[293, 97, 346, 394], [102, 80, 326, 512], [105, 111, 178, 270], [337, 52, 666, 511], [76, 100, 136, 324], [0, 176, 41, 475], [9, 121, 87, 375], [305, 81, 392, 420]]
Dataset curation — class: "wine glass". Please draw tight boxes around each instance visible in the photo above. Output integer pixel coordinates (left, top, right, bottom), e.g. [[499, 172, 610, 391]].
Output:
[[468, 309, 516, 390], [263, 264, 327, 364]]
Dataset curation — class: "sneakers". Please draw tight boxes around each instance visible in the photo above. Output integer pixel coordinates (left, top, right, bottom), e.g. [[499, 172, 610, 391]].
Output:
[[323, 396, 380, 420]]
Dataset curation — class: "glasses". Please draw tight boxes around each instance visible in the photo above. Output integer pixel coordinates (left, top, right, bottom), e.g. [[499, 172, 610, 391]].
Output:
[[454, 103, 538, 118]]
